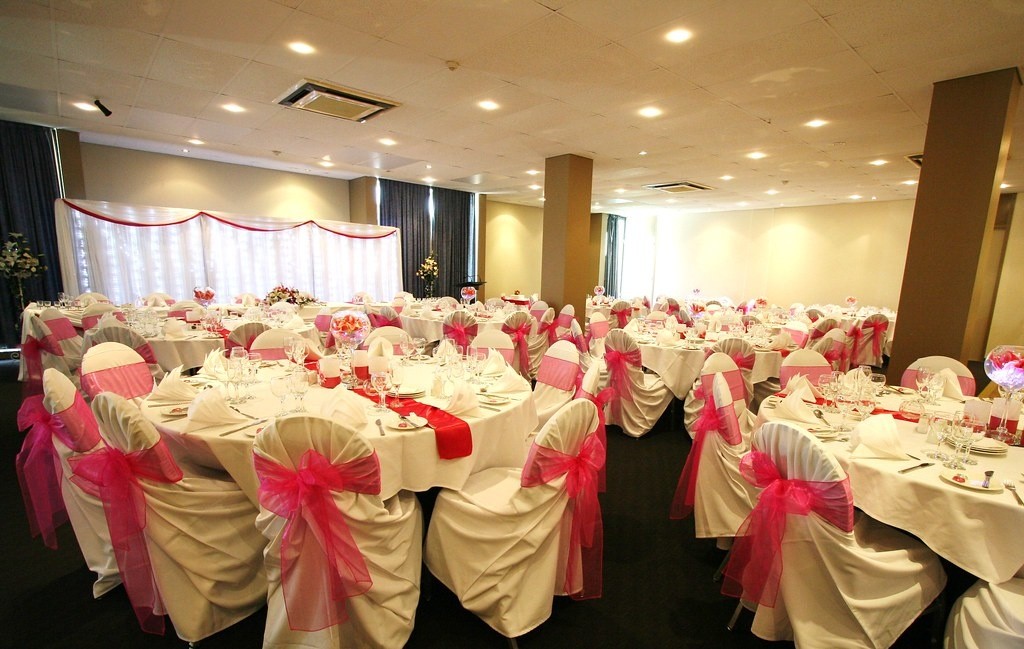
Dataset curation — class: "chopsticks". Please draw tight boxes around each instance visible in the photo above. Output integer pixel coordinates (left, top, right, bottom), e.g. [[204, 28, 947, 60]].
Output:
[[219, 419, 267, 437], [887, 386, 905, 394], [161, 416, 188, 423], [906, 451, 920, 461]]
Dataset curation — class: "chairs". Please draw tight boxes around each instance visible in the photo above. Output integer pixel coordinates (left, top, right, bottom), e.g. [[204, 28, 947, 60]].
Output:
[[0, 292, 1024, 649]]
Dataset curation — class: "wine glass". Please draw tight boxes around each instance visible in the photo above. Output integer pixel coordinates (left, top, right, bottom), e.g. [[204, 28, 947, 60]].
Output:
[[930, 411, 978, 470], [284, 336, 310, 373], [412, 296, 431, 310], [335, 339, 351, 371], [229, 312, 239, 330], [400, 337, 426, 368], [214, 350, 262, 404], [819, 365, 886, 446], [241, 303, 288, 328], [485, 300, 515, 320], [432, 339, 486, 390], [915, 365, 944, 407], [121, 308, 167, 337], [36, 293, 81, 311], [728, 320, 771, 349], [200, 317, 223, 339], [371, 372, 406, 411], [270, 377, 309, 416], [683, 328, 700, 350]]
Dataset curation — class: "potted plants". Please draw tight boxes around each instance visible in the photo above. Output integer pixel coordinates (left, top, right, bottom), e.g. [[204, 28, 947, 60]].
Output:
[[0, 232, 50, 343]]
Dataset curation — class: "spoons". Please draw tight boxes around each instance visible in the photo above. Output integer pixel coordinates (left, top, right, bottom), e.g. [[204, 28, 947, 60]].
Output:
[[813, 409, 832, 428], [982, 470, 994, 488], [399, 415, 419, 428]]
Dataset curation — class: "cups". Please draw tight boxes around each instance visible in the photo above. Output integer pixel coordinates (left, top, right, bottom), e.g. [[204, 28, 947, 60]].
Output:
[[916, 411, 934, 434], [432, 297, 442, 301], [232, 347, 245, 361], [319, 358, 341, 389], [998, 385, 1024, 398], [899, 399, 925, 419], [637, 318, 664, 344], [363, 379, 377, 396], [696, 324, 706, 339], [676, 324, 686, 340]]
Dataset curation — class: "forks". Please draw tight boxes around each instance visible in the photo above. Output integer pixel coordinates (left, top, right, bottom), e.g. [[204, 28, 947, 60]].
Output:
[[1002, 478, 1024, 507], [409, 412, 436, 429]]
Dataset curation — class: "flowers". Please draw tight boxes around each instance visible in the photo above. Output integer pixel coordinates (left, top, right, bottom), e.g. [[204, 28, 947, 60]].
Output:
[[260, 282, 326, 308], [416, 251, 439, 297]]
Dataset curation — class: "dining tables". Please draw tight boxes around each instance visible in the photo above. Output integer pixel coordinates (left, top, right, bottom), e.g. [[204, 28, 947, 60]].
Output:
[[326, 302, 402, 314], [400, 304, 538, 344], [586, 299, 645, 320], [129, 317, 318, 373], [211, 300, 321, 320], [826, 312, 896, 358], [141, 350, 540, 514], [757, 385, 1024, 584], [36, 308, 185, 337], [621, 327, 781, 400]]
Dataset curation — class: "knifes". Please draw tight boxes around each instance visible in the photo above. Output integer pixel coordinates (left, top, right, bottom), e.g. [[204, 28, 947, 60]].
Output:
[[898, 463, 935, 474], [375, 419, 385, 437]]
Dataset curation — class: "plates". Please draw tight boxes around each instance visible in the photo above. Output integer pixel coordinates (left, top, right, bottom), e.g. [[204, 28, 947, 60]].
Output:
[[161, 406, 188, 416], [883, 385, 915, 395], [386, 415, 428, 430], [387, 384, 427, 398], [478, 397, 511, 404], [242, 426, 265, 436], [808, 424, 852, 440], [941, 469, 1005, 491], [947, 432, 1009, 455]]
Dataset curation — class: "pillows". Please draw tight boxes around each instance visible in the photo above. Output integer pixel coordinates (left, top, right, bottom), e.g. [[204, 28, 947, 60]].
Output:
[[434, 340, 462, 366]]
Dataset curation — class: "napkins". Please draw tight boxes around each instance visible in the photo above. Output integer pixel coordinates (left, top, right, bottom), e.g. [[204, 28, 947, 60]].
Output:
[[767, 391, 821, 426], [488, 361, 531, 393], [780, 373, 825, 403], [145, 363, 200, 401], [445, 380, 482, 419], [97, 312, 126, 329], [850, 410, 910, 462], [926, 368, 964, 401], [196, 347, 229, 376], [482, 346, 508, 374], [158, 315, 187, 340], [181, 386, 249, 439], [839, 368, 868, 389], [369, 334, 393, 360], [321, 382, 368, 428]]
